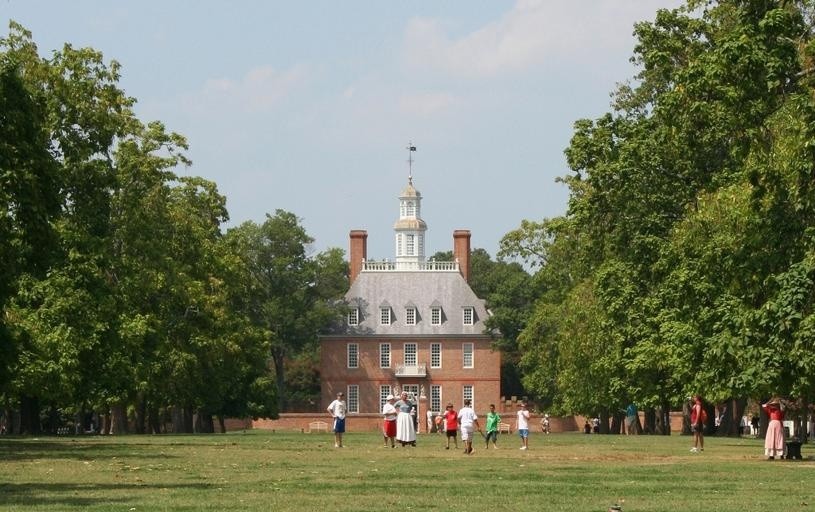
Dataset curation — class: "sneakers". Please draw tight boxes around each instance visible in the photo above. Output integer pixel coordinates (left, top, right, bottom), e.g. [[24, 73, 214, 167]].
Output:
[[690, 447, 704, 453]]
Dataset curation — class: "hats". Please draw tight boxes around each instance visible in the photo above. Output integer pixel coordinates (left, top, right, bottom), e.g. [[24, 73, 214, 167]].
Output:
[[386, 395, 394, 401], [335, 392, 344, 396]]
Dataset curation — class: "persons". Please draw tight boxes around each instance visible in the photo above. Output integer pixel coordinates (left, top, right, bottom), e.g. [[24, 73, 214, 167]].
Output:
[[444, 402, 459, 449], [382, 393, 397, 448], [762, 394, 786, 460], [752, 412, 760, 434], [584, 420, 592, 432], [515, 403, 530, 449], [540, 413, 550, 434], [593, 416, 600, 433], [689, 394, 704, 452], [627, 400, 638, 434], [457, 398, 480, 455], [394, 391, 417, 447], [426, 407, 433, 433], [485, 404, 501, 449], [327, 391, 348, 447], [435, 413, 442, 433], [410, 406, 416, 423]]
